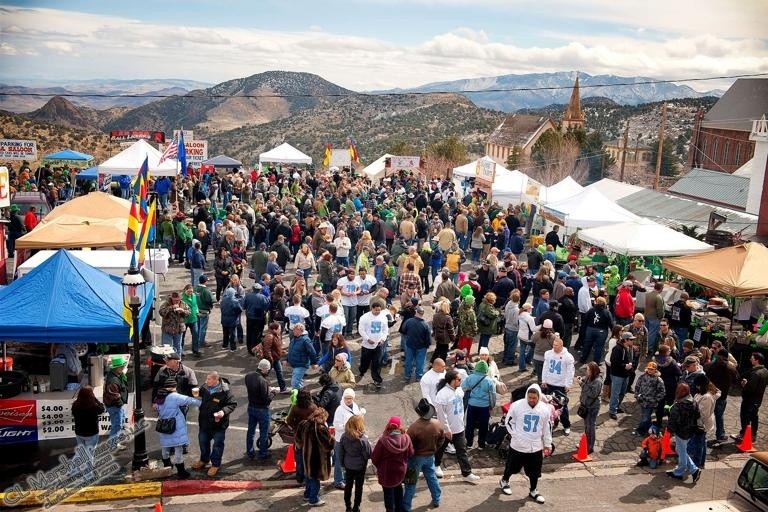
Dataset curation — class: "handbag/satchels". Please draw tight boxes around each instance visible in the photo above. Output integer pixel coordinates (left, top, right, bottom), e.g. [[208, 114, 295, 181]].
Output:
[[155, 418, 177, 435]]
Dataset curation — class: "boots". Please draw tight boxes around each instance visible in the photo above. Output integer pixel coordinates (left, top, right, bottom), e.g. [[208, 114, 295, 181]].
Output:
[[176, 462, 190, 478], [163, 458, 173, 470]]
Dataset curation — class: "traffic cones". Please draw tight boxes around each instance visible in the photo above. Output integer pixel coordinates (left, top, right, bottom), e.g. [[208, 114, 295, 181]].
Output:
[[738, 425, 757, 453], [658, 427, 677, 459], [570, 432, 595, 465], [278, 443, 298, 473]]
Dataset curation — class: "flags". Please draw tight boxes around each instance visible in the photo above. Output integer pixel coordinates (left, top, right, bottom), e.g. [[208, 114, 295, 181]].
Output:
[[158, 132, 178, 166], [322, 143, 331, 167], [348, 138, 360, 164], [177, 128, 188, 178]]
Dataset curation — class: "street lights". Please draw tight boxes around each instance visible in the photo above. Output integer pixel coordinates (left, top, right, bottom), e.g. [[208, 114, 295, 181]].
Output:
[[119, 263, 149, 478]]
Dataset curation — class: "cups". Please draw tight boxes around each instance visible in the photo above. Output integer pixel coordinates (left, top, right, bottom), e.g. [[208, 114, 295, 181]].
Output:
[[328, 426, 336, 433], [214, 411, 221, 422], [192, 387, 200, 398]]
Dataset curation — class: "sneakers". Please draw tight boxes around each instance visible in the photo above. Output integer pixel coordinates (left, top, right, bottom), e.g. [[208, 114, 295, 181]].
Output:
[[191, 317, 666, 434], [308, 501, 326, 507], [634, 433, 756, 467], [206, 465, 220, 476], [335, 482, 346, 489], [529, 490, 545, 504], [499, 480, 513, 496], [666, 470, 685, 480], [192, 460, 208, 469], [434, 466, 444, 479], [692, 468, 701, 484], [463, 473, 480, 482]]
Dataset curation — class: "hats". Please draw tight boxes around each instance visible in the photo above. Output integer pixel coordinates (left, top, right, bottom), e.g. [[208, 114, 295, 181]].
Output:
[[108, 356, 127, 369], [163, 354, 181, 361], [165, 379, 177, 387], [215, 214, 639, 314], [8, 168, 72, 226], [169, 291, 184, 301]]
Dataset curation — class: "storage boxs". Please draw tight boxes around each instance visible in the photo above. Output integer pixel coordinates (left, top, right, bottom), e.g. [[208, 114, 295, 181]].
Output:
[[694, 310, 744, 336]]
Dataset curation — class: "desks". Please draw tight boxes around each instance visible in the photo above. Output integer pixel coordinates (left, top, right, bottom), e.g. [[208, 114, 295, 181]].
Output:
[[689, 325, 729, 349], [0, 388, 136, 443], [144, 248, 172, 283], [537, 244, 592, 266], [72, 352, 132, 398]]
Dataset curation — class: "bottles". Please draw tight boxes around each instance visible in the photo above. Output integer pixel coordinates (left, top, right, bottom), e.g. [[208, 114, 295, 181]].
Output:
[[21, 375, 51, 395]]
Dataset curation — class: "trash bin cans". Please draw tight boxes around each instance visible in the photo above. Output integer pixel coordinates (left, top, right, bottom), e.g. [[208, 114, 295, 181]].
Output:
[[150, 344, 177, 385], [0, 357, 13, 372]]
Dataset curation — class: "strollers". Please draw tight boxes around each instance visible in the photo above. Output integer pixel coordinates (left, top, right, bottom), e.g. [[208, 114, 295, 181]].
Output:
[[257, 388, 299, 452], [488, 389, 570, 461]]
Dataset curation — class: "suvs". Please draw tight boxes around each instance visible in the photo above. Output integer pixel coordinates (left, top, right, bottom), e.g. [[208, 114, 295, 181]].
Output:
[[10, 190, 53, 227], [651, 451, 767, 511]]
